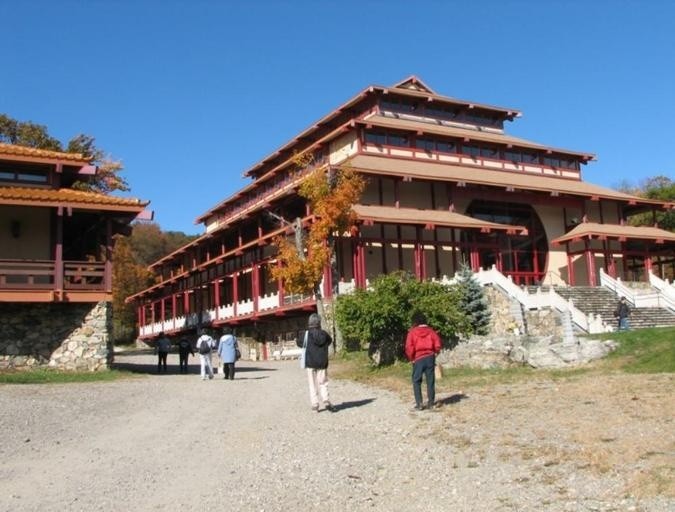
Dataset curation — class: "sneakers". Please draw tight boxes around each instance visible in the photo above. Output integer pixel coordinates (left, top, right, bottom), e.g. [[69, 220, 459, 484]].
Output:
[[310, 401, 334, 412], [415, 403, 435, 410]]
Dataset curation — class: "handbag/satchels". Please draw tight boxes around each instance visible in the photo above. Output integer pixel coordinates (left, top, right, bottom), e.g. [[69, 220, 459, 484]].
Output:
[[236, 350, 240, 359], [299, 347, 307, 368]]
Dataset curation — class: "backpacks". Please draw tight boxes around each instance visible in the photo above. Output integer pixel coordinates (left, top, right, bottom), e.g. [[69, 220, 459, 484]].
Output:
[[200, 341, 210, 354]]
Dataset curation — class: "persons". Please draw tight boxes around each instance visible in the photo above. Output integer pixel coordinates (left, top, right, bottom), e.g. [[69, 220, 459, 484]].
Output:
[[614, 296, 630, 328], [405, 311, 442, 411], [157, 326, 238, 380], [296, 312, 333, 412]]
